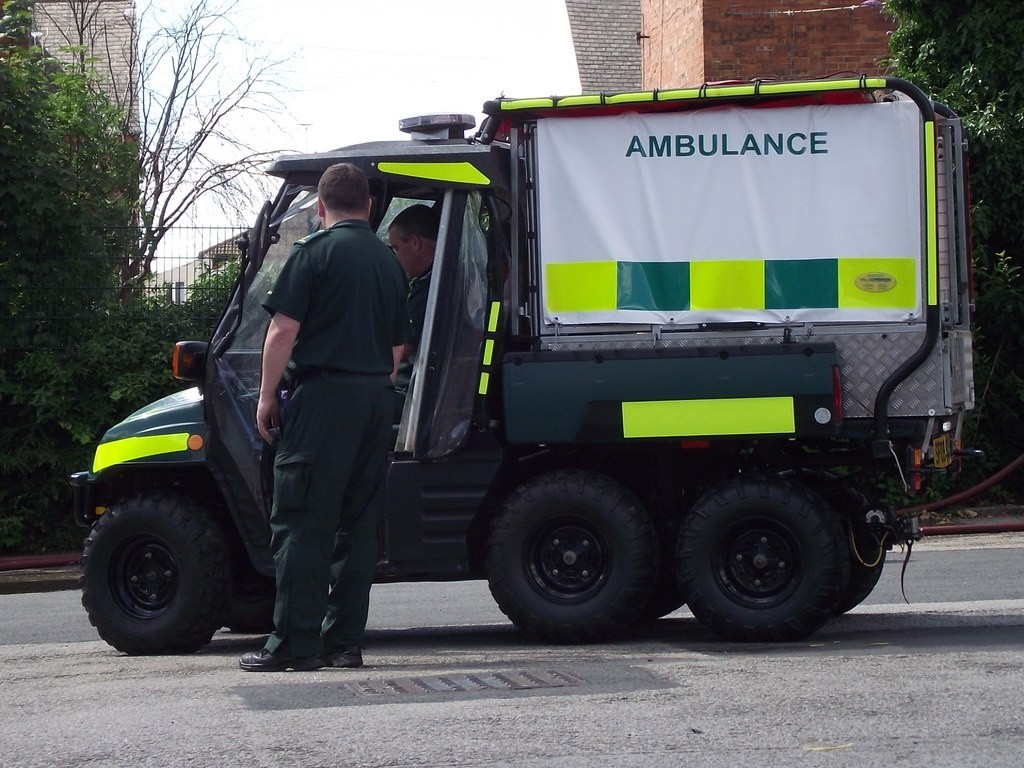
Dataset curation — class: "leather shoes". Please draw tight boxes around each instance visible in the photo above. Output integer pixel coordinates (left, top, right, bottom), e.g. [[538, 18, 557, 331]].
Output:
[[239, 649, 318, 673], [319, 645, 363, 668]]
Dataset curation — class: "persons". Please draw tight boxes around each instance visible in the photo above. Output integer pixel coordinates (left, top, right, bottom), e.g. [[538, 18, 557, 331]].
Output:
[[241, 162, 439, 669]]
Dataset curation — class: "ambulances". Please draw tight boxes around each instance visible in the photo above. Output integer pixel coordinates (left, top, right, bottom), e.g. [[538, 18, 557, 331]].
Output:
[[65, 74, 978, 656]]
